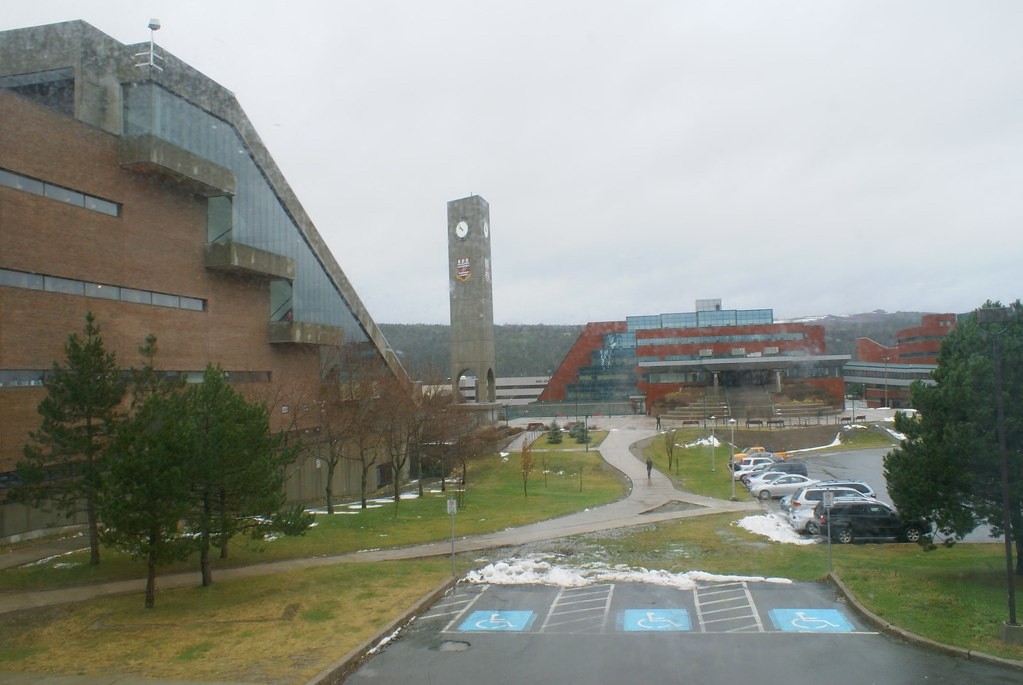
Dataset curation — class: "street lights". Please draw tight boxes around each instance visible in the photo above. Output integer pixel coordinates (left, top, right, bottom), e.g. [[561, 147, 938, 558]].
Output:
[[709, 415, 718, 471], [726, 418, 737, 501], [882, 357, 891, 407]]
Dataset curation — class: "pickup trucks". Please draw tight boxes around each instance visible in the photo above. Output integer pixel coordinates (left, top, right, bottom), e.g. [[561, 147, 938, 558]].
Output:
[[730, 447, 787, 461]]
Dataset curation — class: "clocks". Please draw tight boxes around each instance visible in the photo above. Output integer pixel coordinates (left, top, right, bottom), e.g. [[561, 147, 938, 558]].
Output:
[[456, 221, 469, 237], [483, 222, 488, 238]]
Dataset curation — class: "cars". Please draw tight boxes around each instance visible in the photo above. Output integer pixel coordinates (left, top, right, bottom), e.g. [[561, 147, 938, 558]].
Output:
[[730, 451, 785, 482], [749, 474, 822, 500], [789, 497, 878, 535], [746, 471, 796, 491]]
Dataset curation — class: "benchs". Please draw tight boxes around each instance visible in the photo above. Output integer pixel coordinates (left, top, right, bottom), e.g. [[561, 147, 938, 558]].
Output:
[[746, 421, 763, 427], [854, 416, 866, 423], [767, 421, 784, 427], [683, 421, 699, 427], [838, 417, 852, 424], [528, 423, 543, 430]]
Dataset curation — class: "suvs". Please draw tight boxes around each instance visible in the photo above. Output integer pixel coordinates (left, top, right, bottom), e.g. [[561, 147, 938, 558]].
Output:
[[813, 497, 933, 545], [741, 461, 808, 485], [787, 485, 869, 511], [779, 478, 877, 512]]
